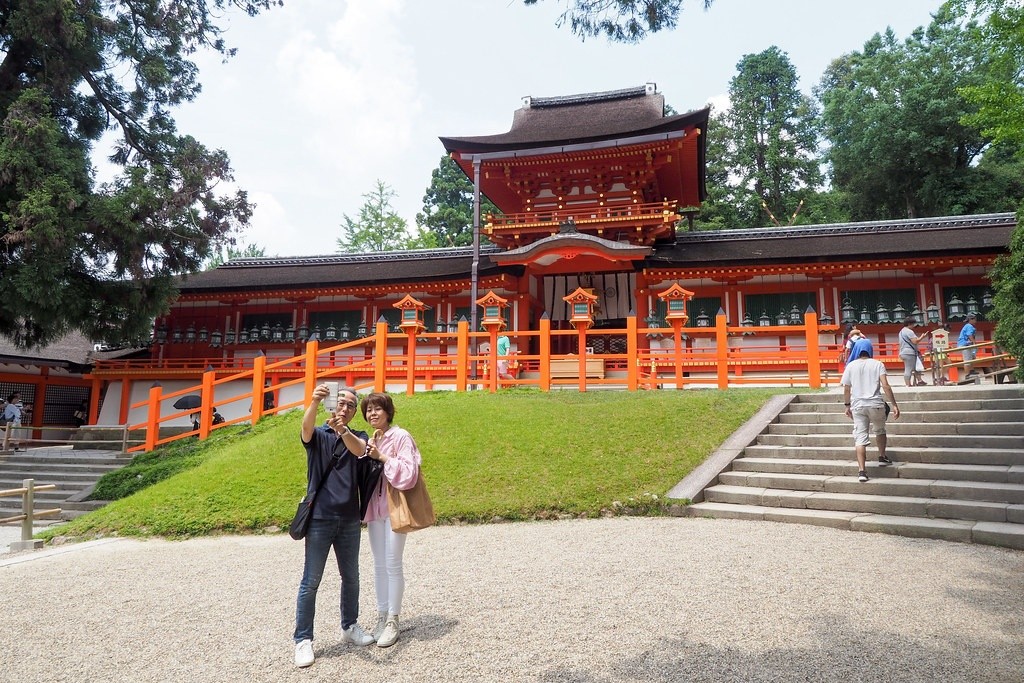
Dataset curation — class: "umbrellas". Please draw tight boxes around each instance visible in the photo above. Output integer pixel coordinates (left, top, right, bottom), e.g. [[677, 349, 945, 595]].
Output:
[[173, 395, 202, 410]]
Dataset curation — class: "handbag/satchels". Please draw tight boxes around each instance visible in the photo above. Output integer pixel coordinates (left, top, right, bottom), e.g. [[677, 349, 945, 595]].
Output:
[[916, 356, 925, 371], [389, 466, 434, 533], [884, 401, 890, 420], [289, 499, 312, 539]]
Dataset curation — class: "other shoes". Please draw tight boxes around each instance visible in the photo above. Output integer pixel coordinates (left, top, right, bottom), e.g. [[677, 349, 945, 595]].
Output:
[[969, 370, 979, 374], [918, 381, 926, 386], [965, 373, 973, 380]]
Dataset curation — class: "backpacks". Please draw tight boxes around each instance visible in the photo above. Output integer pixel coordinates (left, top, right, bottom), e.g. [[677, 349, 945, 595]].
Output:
[[849, 337, 860, 354]]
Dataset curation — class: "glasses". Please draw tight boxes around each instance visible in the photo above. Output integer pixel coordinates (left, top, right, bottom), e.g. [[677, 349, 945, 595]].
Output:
[[336, 400, 358, 411]]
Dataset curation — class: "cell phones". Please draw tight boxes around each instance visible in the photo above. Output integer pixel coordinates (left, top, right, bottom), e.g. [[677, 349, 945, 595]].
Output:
[[323, 382, 338, 412]]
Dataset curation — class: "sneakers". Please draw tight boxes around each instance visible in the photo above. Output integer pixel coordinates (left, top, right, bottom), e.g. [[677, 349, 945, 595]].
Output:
[[295, 640, 315, 668], [377, 616, 399, 647], [857, 471, 869, 482], [877, 456, 893, 466], [342, 622, 373, 647], [372, 616, 388, 641]]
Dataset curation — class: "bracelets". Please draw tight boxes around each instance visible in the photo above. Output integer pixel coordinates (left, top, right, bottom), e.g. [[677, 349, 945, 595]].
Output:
[[337, 426, 349, 437], [845, 403, 850, 406]]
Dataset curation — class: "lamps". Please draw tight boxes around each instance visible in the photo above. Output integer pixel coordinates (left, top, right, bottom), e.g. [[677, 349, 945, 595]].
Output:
[[645, 268, 997, 340], [129, 287, 510, 348]]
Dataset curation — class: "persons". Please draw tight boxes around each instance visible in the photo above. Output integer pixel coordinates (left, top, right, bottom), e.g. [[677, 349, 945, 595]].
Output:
[[2, 395, 24, 451], [359, 394, 422, 648], [0, 391, 29, 428], [899, 316, 928, 387], [76, 400, 88, 428], [292, 387, 374, 669], [843, 323, 867, 370], [188, 409, 201, 438], [212, 407, 225, 426], [248, 379, 275, 418], [840, 340, 900, 481], [497, 328, 519, 390], [957, 313, 980, 381]]
